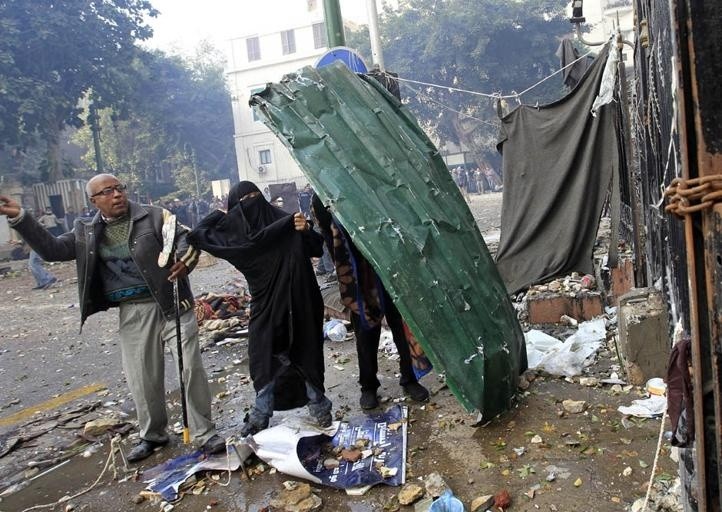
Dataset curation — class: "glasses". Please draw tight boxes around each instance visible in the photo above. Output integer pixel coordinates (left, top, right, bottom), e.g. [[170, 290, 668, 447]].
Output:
[[91, 183, 126, 197]]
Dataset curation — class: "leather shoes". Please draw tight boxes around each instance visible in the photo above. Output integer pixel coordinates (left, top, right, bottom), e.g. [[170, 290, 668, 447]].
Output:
[[317, 411, 335, 426], [127, 439, 168, 462], [403, 381, 430, 402], [240, 419, 266, 439], [360, 388, 378, 409], [198, 436, 226, 455]]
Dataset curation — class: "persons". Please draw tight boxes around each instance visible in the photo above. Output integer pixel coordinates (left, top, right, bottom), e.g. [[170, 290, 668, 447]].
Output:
[[81, 205, 92, 217], [310, 186, 431, 413], [306, 182, 314, 211], [1, 172, 227, 459], [450, 165, 496, 196], [38, 206, 64, 238], [169, 193, 229, 231], [64, 205, 77, 232], [263, 187, 272, 203], [298, 187, 310, 217], [185, 178, 334, 437], [5, 235, 56, 291], [270, 183, 299, 215]]
